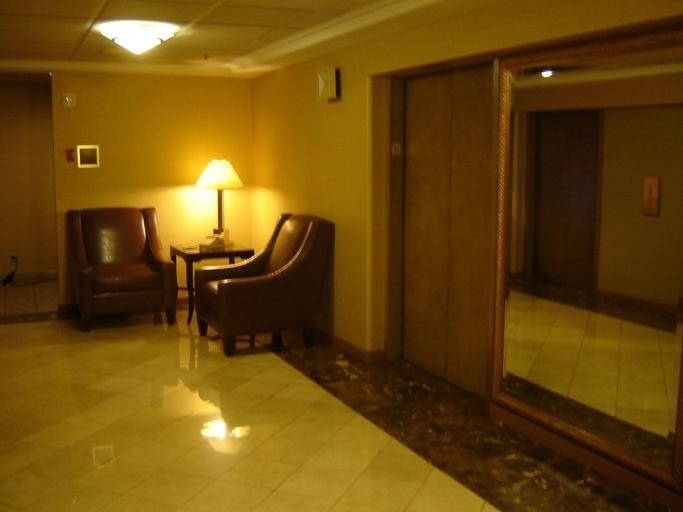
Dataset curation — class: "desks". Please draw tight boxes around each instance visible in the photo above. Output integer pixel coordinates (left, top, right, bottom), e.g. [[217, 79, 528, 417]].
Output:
[[171, 241, 255, 324]]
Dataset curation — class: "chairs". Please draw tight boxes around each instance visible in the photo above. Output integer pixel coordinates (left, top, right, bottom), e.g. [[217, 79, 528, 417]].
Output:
[[194, 212, 335, 358], [65, 204, 176, 332]]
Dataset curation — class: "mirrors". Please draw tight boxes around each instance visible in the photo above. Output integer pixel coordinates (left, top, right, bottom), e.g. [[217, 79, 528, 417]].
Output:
[[494, 64, 683, 512]]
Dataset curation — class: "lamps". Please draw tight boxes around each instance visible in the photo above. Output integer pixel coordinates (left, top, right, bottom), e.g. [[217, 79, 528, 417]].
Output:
[[196, 158, 244, 246]]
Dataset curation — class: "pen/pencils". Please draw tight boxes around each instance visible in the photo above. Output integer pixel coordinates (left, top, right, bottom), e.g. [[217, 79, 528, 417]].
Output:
[[183, 247, 195, 250]]
